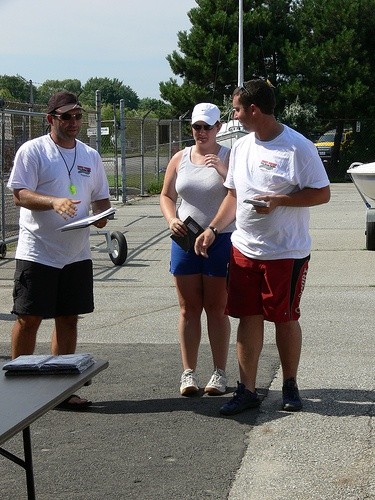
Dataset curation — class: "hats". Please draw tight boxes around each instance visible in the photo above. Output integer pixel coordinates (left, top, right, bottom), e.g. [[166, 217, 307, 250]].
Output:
[[191, 103, 221, 126], [47, 91, 86, 113]]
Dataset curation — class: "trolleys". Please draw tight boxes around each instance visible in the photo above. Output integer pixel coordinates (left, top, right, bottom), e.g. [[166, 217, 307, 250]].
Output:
[[90, 230, 128, 265], [348, 161, 375, 251]]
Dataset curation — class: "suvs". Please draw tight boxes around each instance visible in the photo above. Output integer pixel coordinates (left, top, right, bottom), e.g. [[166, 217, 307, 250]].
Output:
[[313, 128, 354, 173]]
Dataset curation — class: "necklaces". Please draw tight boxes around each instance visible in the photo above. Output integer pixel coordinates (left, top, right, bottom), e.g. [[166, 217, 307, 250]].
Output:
[[49, 133, 77, 195]]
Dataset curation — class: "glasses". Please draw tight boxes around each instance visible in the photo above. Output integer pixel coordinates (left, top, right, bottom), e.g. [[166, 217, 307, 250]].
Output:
[[192, 123, 219, 131], [241, 82, 249, 95], [52, 113, 83, 120]]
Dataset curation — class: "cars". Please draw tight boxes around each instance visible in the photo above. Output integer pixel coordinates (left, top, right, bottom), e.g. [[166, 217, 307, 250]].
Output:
[[108, 129, 154, 154]]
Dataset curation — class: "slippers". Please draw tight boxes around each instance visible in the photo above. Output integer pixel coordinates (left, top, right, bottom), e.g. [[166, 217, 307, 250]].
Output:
[[56, 395, 92, 408]]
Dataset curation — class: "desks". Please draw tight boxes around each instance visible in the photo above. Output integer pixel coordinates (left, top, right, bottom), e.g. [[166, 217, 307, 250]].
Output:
[[0, 359, 110, 500]]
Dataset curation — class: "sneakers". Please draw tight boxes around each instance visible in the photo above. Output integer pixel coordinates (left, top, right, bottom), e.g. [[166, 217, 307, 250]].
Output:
[[220, 380, 261, 415], [179, 368, 199, 395], [281, 377, 303, 411], [204, 365, 227, 394]]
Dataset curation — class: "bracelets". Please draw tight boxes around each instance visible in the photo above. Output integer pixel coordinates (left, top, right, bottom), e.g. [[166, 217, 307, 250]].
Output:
[[209, 226, 217, 235]]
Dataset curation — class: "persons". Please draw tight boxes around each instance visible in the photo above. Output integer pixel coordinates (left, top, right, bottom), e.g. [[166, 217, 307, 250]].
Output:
[[160, 102, 237, 395], [6, 92, 112, 408], [193, 79, 331, 415]]
